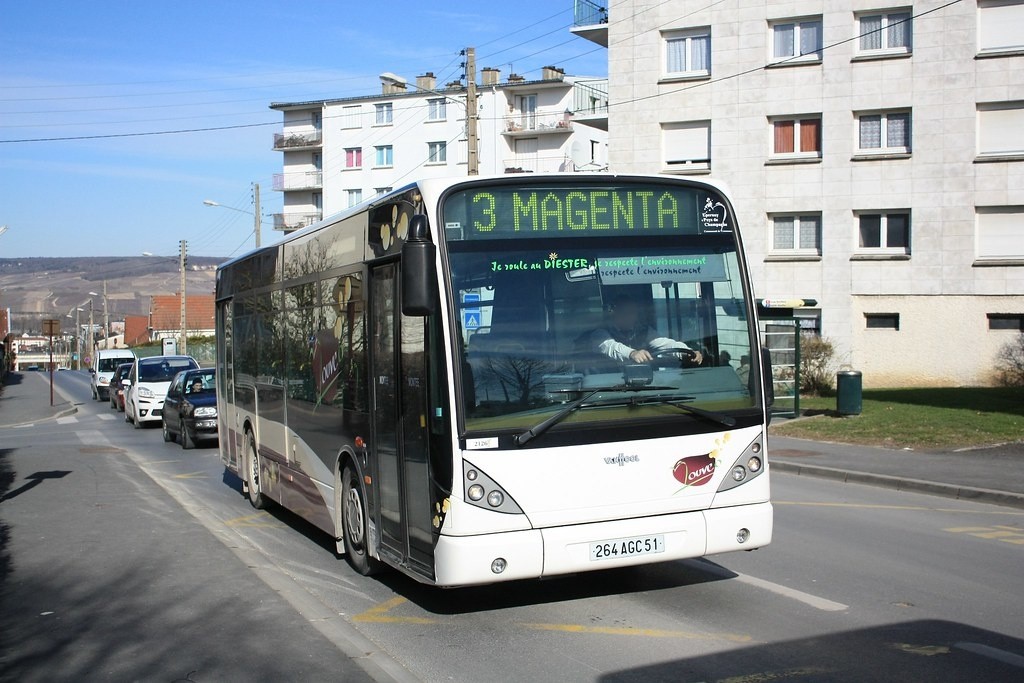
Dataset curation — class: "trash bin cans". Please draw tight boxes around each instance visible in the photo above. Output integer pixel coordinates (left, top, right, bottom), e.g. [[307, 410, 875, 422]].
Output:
[[835, 371, 863, 416]]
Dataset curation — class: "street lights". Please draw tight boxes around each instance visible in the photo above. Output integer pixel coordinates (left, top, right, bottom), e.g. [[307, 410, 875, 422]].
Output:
[[89, 292, 109, 349], [143, 239, 188, 355], [203, 184, 262, 250], [78, 308, 94, 369], [378, 70, 479, 176]]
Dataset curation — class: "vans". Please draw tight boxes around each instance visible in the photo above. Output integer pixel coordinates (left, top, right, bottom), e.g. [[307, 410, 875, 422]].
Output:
[[88, 349, 137, 402]]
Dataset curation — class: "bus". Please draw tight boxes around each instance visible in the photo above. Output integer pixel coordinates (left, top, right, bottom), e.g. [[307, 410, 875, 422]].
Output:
[[216, 162, 776, 591]]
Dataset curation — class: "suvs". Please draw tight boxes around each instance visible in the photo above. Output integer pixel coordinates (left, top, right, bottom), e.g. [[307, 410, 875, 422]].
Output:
[[121, 355, 214, 429]]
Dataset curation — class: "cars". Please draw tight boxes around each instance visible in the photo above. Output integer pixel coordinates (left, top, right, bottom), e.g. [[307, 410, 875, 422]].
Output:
[[28, 365, 38, 371], [109, 363, 134, 412], [57, 365, 67, 371], [161, 367, 219, 450]]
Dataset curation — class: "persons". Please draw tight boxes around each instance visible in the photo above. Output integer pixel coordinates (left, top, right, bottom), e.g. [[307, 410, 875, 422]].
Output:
[[192, 379, 203, 393], [588, 296, 703, 373]]
